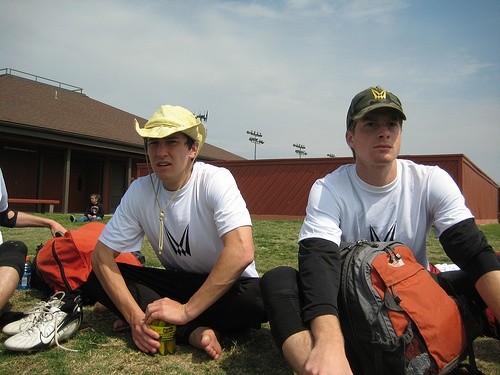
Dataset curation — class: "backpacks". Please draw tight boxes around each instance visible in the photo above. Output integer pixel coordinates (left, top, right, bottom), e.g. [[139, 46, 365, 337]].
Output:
[[30, 222, 144, 294], [340, 239, 480, 375]]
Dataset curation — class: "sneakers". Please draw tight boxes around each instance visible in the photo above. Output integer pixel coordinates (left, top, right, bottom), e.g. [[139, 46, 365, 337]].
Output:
[[3, 298, 83, 352]]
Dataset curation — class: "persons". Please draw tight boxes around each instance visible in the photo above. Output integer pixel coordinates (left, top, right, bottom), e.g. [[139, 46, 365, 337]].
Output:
[[260, 87, 500, 375], [70, 193, 104, 222], [86, 105, 268, 359], [0, 168, 67, 311]]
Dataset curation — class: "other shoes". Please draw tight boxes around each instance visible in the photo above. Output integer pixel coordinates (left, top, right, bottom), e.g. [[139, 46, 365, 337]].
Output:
[[70, 215, 75, 222]]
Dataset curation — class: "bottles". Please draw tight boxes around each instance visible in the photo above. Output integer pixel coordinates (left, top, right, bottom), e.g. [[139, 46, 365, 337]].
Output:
[[15, 256, 32, 291]]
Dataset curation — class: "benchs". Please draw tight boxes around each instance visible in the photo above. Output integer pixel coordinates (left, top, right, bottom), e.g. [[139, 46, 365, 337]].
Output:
[[8, 197, 60, 213]]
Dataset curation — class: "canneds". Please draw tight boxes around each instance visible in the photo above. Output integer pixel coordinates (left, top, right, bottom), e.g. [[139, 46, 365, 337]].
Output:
[[147, 319, 176, 354]]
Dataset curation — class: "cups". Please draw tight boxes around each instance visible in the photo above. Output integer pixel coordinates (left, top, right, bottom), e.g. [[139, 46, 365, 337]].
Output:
[[147, 319, 176, 356]]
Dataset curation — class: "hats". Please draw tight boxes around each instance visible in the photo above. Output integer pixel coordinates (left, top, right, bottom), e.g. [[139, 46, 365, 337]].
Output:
[[133, 105, 206, 158], [346, 86, 406, 128]]
